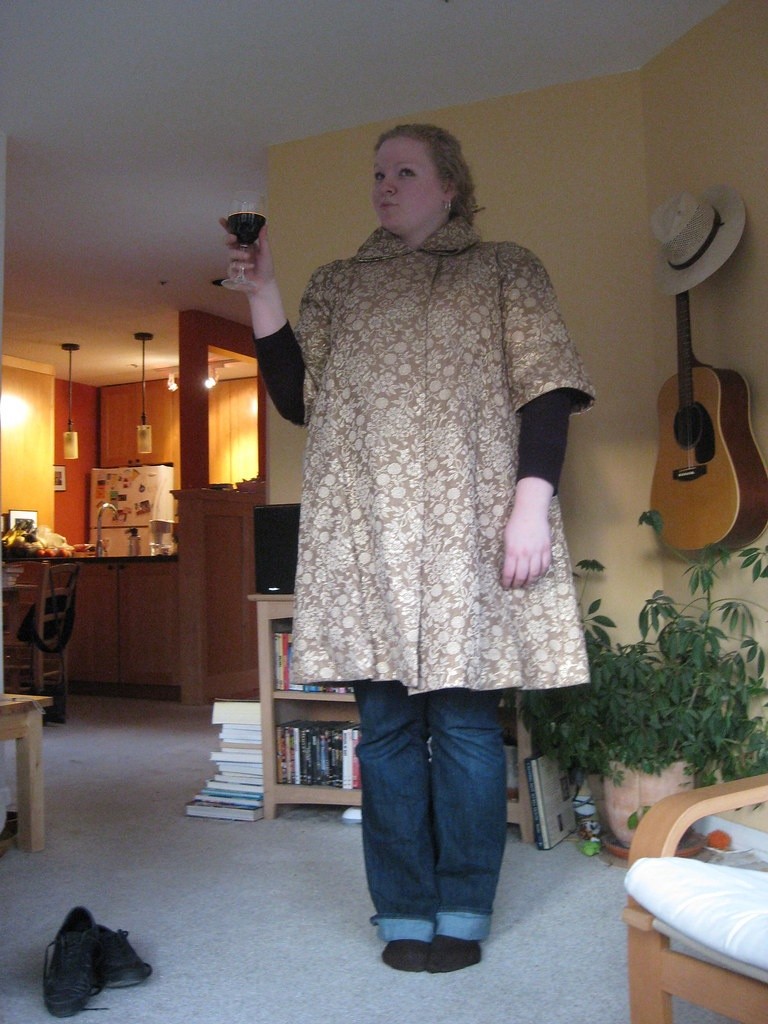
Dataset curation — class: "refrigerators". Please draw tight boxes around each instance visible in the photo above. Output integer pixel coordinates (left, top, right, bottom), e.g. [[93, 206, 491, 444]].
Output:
[[87, 464, 174, 556]]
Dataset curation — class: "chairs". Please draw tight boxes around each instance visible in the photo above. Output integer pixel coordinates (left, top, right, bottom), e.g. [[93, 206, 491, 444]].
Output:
[[620, 772, 767, 1024], [0, 693, 53, 853], [3, 560, 80, 699]]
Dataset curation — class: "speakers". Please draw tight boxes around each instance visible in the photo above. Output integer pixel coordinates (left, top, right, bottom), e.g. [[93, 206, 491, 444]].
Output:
[[254, 503, 301, 594]]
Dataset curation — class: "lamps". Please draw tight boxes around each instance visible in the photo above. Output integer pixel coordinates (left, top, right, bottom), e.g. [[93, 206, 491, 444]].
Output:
[[205, 368, 219, 388], [167, 374, 179, 393], [61, 343, 79, 459], [133, 332, 154, 453]]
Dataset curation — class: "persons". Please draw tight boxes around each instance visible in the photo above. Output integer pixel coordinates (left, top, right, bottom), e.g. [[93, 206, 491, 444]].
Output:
[[219, 121, 597, 976]]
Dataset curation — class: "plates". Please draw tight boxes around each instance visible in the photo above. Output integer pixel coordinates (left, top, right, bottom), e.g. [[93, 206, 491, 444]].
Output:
[[209, 484, 233, 489]]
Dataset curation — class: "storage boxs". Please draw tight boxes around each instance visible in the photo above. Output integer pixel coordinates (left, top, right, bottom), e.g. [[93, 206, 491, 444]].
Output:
[[524, 753, 578, 850]]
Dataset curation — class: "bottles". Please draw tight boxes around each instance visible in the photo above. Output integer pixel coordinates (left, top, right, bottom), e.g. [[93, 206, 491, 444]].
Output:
[[125, 527, 141, 556], [150, 541, 167, 556]]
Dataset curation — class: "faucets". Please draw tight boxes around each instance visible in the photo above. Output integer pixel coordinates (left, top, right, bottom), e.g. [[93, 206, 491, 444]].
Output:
[[96, 503, 117, 557]]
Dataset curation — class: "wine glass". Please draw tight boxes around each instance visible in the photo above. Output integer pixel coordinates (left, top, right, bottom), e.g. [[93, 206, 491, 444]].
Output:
[[221, 189, 266, 291]]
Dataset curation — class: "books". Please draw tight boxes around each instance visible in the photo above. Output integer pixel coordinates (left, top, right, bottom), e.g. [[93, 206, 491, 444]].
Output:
[[182, 634, 581, 851]]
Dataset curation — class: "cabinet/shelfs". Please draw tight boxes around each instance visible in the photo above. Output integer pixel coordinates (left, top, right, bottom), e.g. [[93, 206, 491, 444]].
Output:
[[46, 563, 182, 701], [247, 594, 539, 844], [100, 379, 258, 555]]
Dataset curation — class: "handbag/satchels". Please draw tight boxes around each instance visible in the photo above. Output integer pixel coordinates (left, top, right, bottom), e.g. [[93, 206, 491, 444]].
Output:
[[18, 594, 66, 643]]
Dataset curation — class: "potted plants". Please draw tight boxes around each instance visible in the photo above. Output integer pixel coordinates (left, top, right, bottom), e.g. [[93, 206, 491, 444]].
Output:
[[513, 510, 768, 852]]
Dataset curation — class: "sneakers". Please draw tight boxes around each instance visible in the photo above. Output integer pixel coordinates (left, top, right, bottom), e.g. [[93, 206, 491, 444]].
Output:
[[94, 922, 151, 988], [39, 904, 102, 1019]]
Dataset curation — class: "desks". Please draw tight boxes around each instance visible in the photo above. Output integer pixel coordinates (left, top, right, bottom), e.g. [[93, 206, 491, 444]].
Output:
[[3, 584, 38, 694]]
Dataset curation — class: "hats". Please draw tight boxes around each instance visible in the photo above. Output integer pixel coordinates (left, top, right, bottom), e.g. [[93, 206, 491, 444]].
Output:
[[640, 184, 745, 294]]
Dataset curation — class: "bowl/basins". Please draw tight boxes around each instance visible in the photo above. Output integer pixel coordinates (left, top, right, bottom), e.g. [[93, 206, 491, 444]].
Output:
[[236, 482, 258, 493]]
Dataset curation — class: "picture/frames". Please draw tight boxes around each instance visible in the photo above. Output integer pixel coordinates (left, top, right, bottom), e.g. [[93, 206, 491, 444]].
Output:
[[54, 464, 67, 492]]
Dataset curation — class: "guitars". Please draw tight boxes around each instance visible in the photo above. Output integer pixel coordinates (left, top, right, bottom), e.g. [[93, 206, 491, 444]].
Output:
[[648, 291, 768, 557]]
